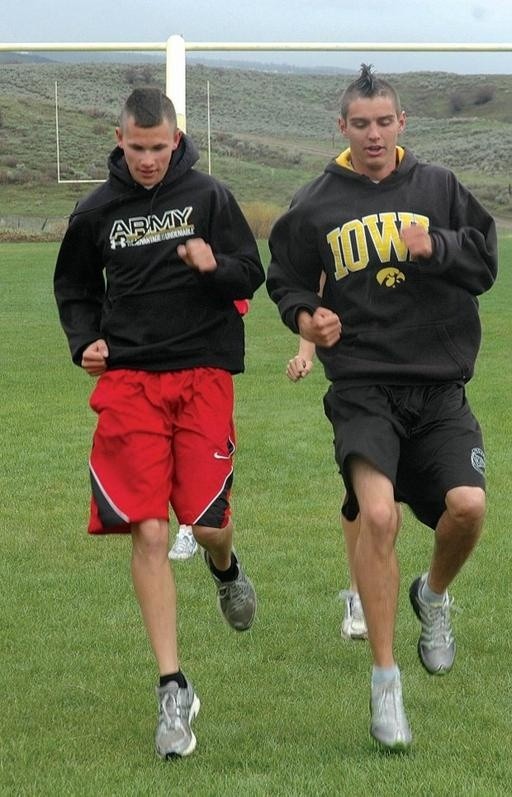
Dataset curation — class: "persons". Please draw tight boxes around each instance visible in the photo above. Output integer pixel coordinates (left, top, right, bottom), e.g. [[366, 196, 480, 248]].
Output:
[[284, 267, 366, 638], [165, 298, 250, 559], [264, 61, 499, 749], [50, 88, 264, 756]]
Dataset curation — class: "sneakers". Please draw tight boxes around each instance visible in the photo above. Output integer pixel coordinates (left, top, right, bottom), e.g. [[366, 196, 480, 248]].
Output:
[[338, 583, 368, 639], [200, 544, 257, 630], [168, 524, 198, 560], [154, 668, 201, 758], [370, 663, 412, 751], [409, 573, 463, 675]]
